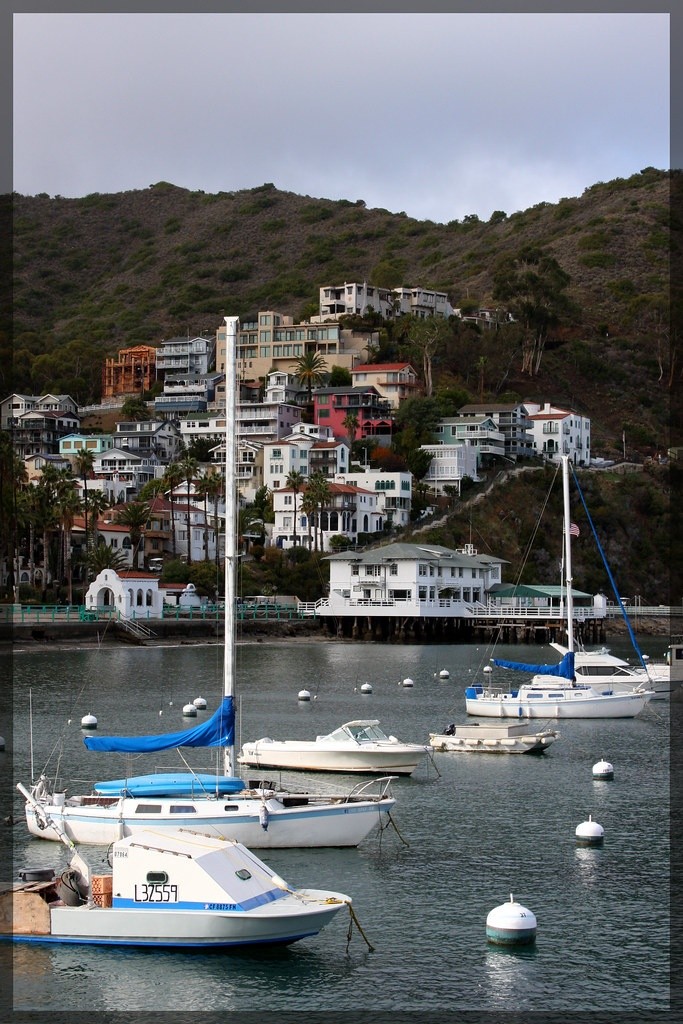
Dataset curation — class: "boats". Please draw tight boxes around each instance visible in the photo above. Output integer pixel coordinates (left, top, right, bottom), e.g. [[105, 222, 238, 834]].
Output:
[[237, 718, 436, 776], [548, 629, 683, 693], [0, 780, 354, 949], [427, 719, 561, 754]]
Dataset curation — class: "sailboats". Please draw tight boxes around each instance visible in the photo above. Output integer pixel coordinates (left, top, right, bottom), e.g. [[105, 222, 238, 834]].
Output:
[[13, 313, 402, 854], [462, 454, 656, 719]]
